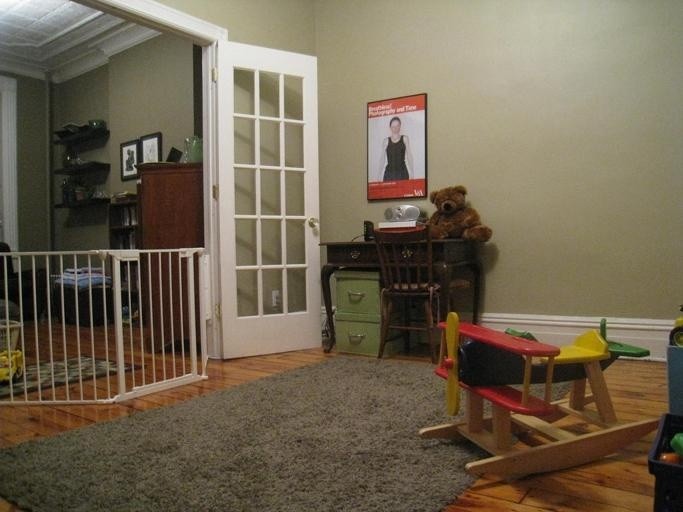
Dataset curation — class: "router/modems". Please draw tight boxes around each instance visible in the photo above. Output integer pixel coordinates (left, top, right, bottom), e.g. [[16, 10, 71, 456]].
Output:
[[364, 220, 374, 241]]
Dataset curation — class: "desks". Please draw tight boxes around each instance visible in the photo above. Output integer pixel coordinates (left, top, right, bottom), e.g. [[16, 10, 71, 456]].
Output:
[[318, 238, 480, 357]]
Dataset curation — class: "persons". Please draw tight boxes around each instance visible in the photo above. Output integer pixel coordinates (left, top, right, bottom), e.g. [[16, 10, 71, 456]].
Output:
[[376, 116, 414, 182]]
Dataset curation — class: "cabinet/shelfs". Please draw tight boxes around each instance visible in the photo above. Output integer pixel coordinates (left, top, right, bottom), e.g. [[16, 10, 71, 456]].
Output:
[[108, 200, 140, 294], [54, 128, 110, 208], [333, 270, 419, 356]]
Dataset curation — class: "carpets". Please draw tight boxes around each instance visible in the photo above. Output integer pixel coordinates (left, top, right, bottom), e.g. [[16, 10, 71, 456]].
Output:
[[1, 354, 580, 512]]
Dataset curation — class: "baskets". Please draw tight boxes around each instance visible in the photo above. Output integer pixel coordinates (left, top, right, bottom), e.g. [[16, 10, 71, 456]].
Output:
[[0, 319, 21, 356]]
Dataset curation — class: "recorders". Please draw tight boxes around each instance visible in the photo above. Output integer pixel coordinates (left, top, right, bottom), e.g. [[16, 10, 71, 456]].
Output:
[[384, 204, 420, 221]]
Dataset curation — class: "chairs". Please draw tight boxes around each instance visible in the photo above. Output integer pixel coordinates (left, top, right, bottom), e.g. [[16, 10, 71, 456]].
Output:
[[0, 242, 46, 321], [372, 223, 442, 364]]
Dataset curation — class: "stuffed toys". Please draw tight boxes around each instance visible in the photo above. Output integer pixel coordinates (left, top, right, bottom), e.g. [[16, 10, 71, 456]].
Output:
[[0, 350, 24, 385], [425, 185, 493, 242]]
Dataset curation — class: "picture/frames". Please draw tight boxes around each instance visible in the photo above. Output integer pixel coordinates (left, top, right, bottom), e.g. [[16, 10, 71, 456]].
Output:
[[367, 93, 428, 202], [120, 131, 163, 180]]
